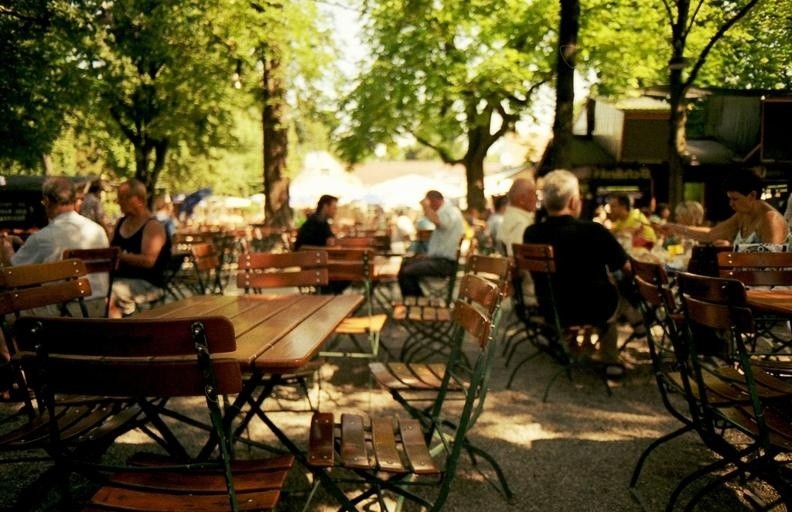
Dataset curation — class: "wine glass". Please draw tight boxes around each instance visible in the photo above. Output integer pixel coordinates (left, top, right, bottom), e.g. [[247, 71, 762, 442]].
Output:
[[640, 236, 656, 258]]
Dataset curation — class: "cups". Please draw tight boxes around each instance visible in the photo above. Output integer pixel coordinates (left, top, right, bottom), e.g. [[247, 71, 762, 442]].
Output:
[[668, 245, 684, 264]]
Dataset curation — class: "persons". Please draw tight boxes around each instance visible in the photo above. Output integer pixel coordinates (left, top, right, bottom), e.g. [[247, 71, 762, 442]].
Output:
[[398, 190, 470, 302], [474, 168, 792, 372], [293, 195, 353, 295], [0, 177, 175, 403]]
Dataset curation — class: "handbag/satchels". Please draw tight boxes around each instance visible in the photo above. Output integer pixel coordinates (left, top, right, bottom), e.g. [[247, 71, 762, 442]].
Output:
[[688, 246, 720, 276]]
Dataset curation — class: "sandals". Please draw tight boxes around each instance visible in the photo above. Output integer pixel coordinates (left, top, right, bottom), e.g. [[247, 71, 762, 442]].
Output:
[[605, 362, 626, 380], [631, 321, 646, 338]]
